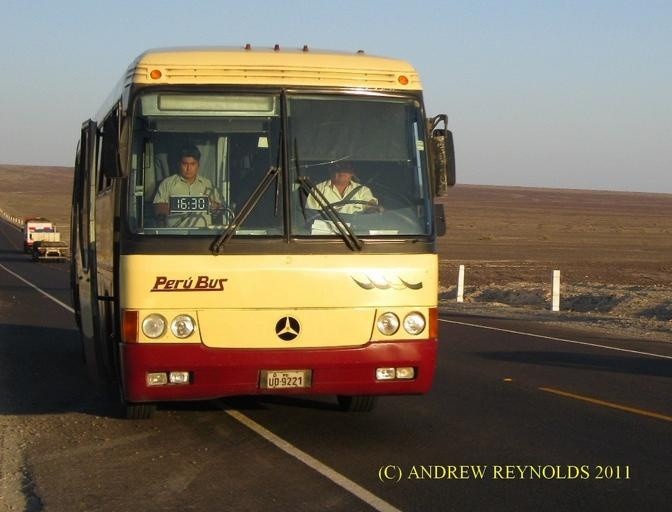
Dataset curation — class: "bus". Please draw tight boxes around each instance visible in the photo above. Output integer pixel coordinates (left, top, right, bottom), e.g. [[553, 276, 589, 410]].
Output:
[[70, 43, 456, 420]]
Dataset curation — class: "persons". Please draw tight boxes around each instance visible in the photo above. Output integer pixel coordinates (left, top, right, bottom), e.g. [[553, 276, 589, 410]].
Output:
[[151, 142, 226, 228], [301, 150, 386, 214]]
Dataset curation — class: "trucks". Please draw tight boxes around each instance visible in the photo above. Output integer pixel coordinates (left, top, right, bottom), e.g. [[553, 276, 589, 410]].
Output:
[[21, 217, 67, 263]]
